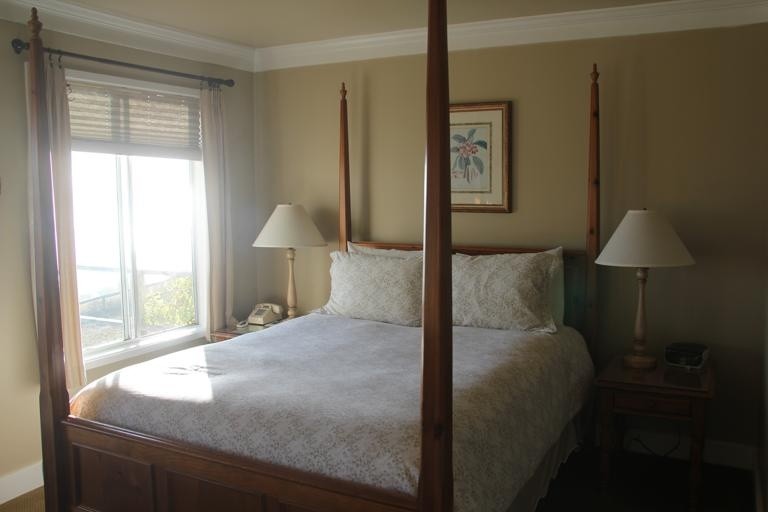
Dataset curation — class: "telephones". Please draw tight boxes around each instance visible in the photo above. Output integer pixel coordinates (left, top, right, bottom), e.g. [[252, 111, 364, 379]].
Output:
[[247, 303, 284, 325]]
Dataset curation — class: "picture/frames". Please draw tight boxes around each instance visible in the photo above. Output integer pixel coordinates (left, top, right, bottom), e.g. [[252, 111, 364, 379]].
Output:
[[449, 100, 511, 213]]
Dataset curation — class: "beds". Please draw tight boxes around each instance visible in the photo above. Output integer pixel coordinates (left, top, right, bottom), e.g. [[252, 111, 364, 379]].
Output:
[[25, 0, 600, 512]]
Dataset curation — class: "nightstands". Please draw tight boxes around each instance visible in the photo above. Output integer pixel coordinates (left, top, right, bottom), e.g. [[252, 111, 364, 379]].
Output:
[[211, 318, 270, 338], [598, 349, 714, 464]]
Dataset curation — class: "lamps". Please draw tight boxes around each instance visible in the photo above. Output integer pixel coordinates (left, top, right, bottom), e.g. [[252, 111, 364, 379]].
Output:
[[251, 204, 328, 318], [594, 210, 695, 367]]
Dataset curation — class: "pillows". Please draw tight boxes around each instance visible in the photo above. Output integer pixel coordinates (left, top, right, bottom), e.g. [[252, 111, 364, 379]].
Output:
[[325, 240, 566, 334]]
